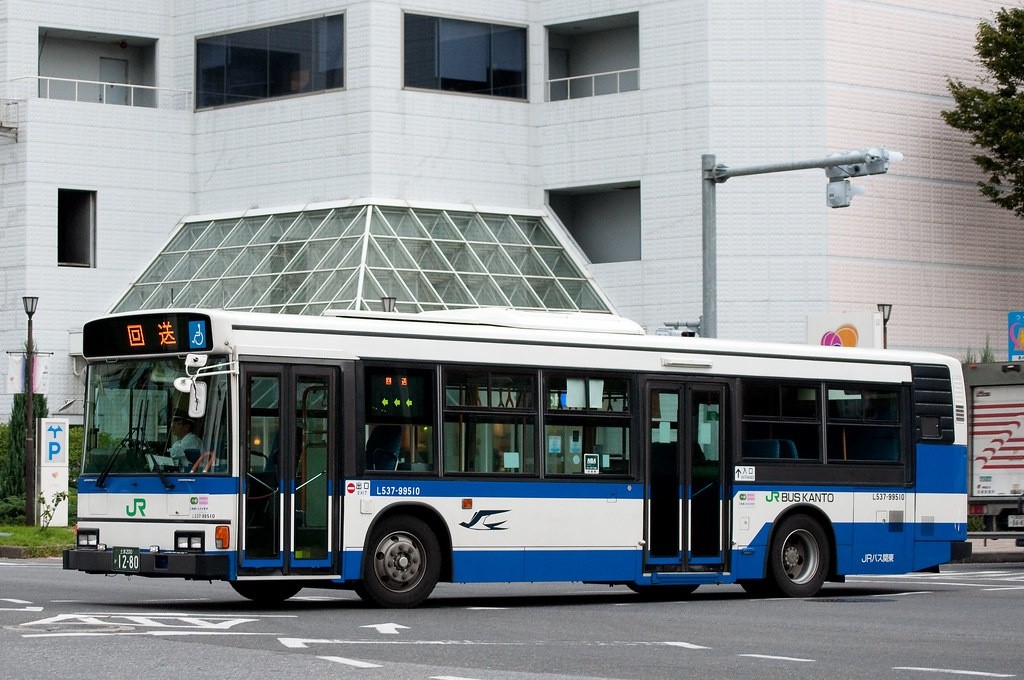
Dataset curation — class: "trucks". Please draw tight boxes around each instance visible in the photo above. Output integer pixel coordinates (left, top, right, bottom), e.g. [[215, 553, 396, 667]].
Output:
[[961, 360, 1024, 547]]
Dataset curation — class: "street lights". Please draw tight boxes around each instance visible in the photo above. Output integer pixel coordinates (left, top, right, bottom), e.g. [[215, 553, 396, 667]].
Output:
[[20, 296, 39, 526], [877, 303, 892, 349]]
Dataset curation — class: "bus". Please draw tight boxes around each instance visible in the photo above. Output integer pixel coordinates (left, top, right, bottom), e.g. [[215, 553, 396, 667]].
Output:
[[63, 307, 969, 610]]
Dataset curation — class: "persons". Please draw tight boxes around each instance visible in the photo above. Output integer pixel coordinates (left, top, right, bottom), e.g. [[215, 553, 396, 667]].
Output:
[[166, 408, 203, 472]]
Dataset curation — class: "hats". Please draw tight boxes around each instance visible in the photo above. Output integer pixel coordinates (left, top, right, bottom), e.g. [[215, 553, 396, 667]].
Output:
[[172, 409, 196, 424]]
